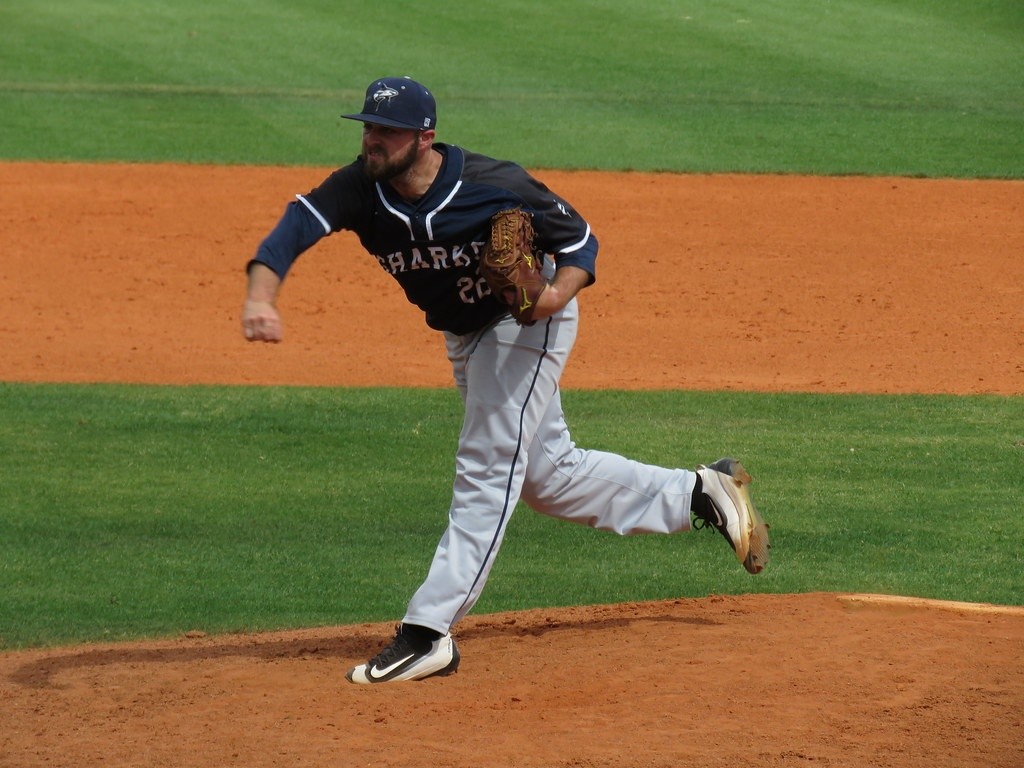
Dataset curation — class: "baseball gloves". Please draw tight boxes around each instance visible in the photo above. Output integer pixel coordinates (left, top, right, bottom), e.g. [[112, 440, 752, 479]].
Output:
[[486, 208, 553, 326]]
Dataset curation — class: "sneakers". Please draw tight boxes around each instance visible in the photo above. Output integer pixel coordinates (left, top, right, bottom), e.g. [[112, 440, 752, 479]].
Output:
[[344, 623, 461, 685], [696, 458, 772, 573]]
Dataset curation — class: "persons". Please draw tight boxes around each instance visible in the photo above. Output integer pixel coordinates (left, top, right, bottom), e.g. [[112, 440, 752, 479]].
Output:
[[243, 74, 772, 685]]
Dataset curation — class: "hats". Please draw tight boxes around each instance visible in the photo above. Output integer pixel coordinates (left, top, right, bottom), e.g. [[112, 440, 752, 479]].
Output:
[[339, 75, 437, 130]]
